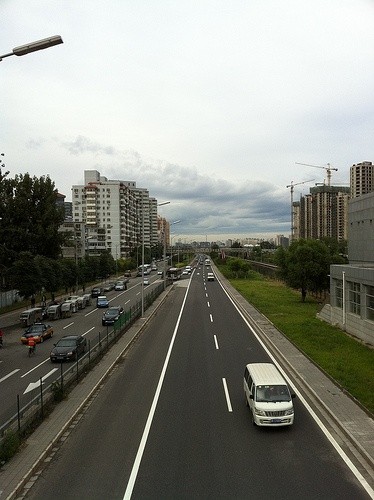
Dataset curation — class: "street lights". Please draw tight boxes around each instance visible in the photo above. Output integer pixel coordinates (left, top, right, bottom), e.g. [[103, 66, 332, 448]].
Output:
[[163, 219, 181, 288], [73, 222, 96, 296], [140, 197, 170, 317]]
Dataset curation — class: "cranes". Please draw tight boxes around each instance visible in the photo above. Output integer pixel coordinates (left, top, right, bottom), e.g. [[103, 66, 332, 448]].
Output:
[[286, 179, 316, 238], [295, 162, 350, 191]]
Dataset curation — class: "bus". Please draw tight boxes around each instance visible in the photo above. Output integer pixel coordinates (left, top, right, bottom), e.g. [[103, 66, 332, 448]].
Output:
[[166, 268, 182, 281]]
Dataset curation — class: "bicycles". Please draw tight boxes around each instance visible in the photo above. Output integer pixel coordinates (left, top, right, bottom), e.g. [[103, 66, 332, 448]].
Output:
[[28, 343, 36, 358]]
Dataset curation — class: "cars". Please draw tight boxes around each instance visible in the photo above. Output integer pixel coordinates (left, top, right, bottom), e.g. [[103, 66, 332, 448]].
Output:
[[96, 295, 110, 309], [207, 272, 215, 281], [50, 334, 87, 363], [182, 270, 189, 275], [20, 323, 53, 345], [140, 277, 151, 287], [17, 251, 169, 330], [102, 305, 125, 326]]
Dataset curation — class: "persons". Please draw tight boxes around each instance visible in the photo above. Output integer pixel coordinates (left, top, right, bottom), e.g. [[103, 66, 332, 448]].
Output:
[[42, 295, 46, 306], [28, 335, 36, 352]]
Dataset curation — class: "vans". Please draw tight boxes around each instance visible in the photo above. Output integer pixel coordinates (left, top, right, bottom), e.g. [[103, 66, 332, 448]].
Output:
[[243, 362, 296, 429]]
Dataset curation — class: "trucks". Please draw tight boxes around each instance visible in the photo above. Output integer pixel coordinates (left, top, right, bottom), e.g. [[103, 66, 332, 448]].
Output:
[[186, 266, 193, 272], [204, 259, 211, 266]]
